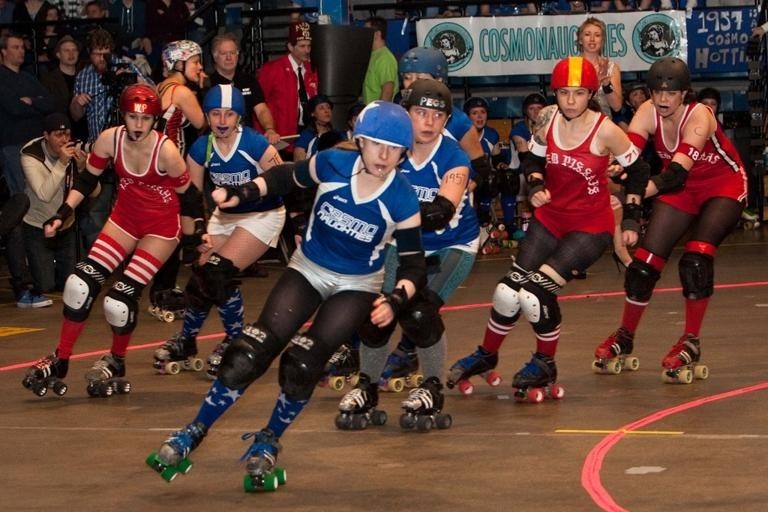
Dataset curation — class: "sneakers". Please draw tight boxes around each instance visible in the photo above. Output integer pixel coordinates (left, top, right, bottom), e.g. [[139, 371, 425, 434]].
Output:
[[17, 288, 53, 308]]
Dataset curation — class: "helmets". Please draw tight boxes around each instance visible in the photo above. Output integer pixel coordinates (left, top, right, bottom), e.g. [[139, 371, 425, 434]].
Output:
[[522, 56, 598, 117], [624, 57, 690, 102], [308, 94, 334, 114], [464, 97, 489, 114], [351, 47, 451, 153]]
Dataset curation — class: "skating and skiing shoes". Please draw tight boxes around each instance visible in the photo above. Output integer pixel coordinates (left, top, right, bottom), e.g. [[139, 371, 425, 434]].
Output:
[[243, 428, 287, 492], [482, 223, 526, 254], [146, 422, 207, 482], [592, 327, 639, 374], [148, 286, 188, 323], [661, 333, 708, 384], [736, 208, 760, 231]]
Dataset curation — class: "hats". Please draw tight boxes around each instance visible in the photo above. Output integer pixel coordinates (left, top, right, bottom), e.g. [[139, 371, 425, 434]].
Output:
[[119, 40, 245, 120], [45, 113, 72, 131], [289, 21, 312, 44]]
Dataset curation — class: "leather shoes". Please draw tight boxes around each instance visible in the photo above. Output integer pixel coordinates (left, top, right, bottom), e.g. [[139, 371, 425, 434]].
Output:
[[233, 263, 268, 278]]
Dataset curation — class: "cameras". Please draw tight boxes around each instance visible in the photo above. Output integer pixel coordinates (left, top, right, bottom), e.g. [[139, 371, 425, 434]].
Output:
[[66, 140, 95, 154]]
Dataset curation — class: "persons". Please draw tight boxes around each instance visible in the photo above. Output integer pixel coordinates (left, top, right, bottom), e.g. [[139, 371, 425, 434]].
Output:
[[151, 84, 287, 376], [156, 99, 429, 480], [603, 55, 748, 383], [447, 56, 649, 411], [325, 78, 480, 432], [361, 1, 768, 280], [2, 1, 487, 324], [19, 85, 207, 401]]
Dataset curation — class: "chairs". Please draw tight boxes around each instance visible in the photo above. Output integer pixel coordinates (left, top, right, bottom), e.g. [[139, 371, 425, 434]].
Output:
[[486, 118, 530, 229]]
